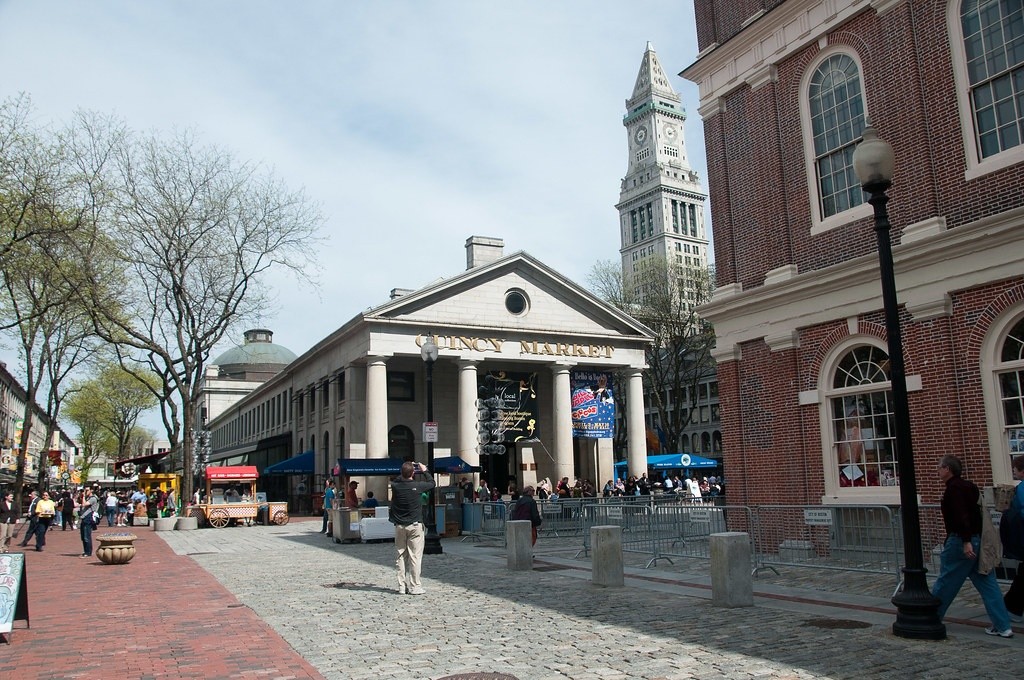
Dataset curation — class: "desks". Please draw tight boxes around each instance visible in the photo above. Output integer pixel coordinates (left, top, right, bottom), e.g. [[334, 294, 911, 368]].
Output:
[[332, 502, 448, 543]]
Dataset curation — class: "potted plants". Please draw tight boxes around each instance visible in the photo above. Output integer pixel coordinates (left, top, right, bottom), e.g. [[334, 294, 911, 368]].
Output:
[[95, 533, 138, 563]]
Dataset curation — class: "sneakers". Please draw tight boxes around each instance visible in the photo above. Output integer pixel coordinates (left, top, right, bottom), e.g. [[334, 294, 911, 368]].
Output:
[[985, 624, 1014, 638]]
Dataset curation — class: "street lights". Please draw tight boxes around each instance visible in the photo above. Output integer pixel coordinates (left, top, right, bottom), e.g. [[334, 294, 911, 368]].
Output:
[[60, 471, 70, 490], [852, 116, 947, 640], [421, 331, 443, 554]]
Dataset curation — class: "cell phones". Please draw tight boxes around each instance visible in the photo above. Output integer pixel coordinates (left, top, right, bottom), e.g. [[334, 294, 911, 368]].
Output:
[[414, 463, 419, 468]]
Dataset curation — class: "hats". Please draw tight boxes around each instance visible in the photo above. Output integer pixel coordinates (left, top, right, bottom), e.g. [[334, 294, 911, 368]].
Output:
[[350, 481, 360, 485]]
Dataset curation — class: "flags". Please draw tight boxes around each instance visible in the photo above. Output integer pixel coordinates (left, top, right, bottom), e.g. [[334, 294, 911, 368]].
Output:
[[48, 451, 61, 467]]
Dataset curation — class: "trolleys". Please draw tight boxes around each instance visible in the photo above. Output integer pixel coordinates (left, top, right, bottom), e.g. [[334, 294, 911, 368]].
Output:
[[194, 466, 257, 529]]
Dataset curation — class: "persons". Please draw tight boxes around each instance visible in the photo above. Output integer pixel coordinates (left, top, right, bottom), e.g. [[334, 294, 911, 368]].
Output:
[[345, 480, 363, 544], [655, 472, 725, 503], [1002, 454, 1024, 562], [0, 491, 55, 551], [194, 484, 252, 526], [508, 479, 542, 559], [455, 477, 507, 523], [388, 461, 436, 595], [536, 473, 653, 520], [363, 491, 379, 508], [319, 479, 341, 537], [100, 486, 178, 526], [55, 483, 100, 558], [837, 406, 886, 464], [931, 454, 1014, 637]]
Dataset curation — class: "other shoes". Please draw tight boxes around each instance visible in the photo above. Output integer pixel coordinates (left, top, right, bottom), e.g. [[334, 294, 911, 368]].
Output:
[[79, 553, 92, 557], [17, 542, 26, 546], [122, 523, 126, 527], [117, 524, 122, 526], [5, 550, 9, 553], [411, 588, 427, 595], [39, 545, 46, 551], [399, 589, 407, 594], [320, 529, 327, 533], [1008, 611, 1023, 622]]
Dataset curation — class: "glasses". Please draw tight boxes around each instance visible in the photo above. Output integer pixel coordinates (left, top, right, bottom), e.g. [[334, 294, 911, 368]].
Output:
[[937, 465, 949, 470]]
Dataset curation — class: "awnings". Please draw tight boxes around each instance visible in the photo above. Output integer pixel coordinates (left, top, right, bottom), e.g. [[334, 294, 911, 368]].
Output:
[[263, 449, 315, 475]]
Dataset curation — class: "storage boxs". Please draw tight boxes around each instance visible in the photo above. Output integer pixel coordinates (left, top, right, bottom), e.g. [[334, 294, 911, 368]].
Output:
[[995, 480, 1022, 511]]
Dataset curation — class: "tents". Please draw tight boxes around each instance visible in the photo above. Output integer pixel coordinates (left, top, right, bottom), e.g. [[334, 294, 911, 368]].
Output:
[[614, 453, 718, 470]]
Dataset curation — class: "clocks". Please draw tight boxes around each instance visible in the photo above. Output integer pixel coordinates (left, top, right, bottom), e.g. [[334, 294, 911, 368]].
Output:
[[634, 125, 647, 145], [663, 124, 678, 141]]
